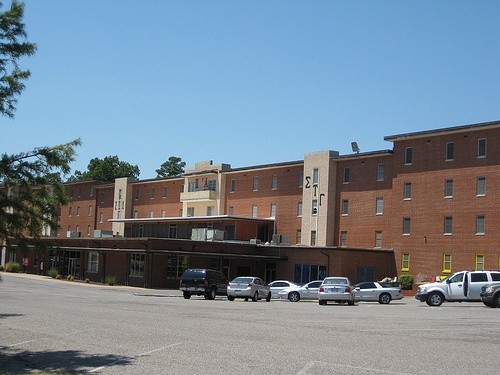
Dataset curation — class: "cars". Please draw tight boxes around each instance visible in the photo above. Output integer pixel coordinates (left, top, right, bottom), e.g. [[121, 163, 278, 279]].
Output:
[[317, 277, 354, 306], [352, 282, 403, 304], [268, 280, 301, 299], [226, 276, 271, 302], [278, 280, 323, 302]]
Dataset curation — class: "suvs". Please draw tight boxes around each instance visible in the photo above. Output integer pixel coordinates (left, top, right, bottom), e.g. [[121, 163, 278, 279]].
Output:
[[180, 269, 230, 300], [479, 283, 500, 308], [415, 270, 500, 306]]
